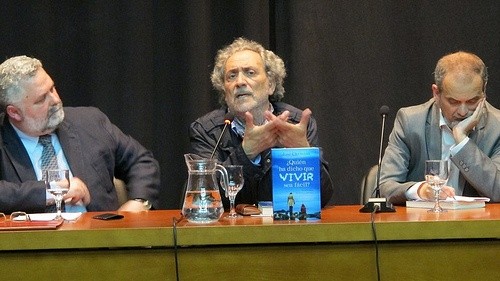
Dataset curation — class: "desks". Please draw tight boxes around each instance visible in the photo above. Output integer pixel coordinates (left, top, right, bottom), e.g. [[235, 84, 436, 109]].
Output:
[[0, 202, 500, 281]]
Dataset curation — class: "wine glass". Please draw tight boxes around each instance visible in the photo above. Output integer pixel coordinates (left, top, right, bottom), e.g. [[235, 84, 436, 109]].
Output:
[[221, 165, 244, 219], [46, 169, 70, 225], [424, 159, 450, 212]]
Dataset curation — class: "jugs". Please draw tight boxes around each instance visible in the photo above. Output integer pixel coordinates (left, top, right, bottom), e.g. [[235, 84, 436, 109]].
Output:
[[182, 153, 229, 223]]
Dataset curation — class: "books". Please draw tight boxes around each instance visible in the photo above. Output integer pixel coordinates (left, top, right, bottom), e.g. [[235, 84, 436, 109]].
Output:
[[406, 196, 486, 210], [257, 202, 273, 215], [271, 147, 321, 220]]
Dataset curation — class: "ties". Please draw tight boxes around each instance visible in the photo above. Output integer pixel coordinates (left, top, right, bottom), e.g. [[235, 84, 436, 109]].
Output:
[[38, 134, 66, 213]]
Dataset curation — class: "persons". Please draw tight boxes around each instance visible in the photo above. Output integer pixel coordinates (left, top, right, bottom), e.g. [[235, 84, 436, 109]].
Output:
[[0, 55, 161, 214], [372, 51, 500, 206], [287, 193, 307, 218], [181, 37, 334, 212]]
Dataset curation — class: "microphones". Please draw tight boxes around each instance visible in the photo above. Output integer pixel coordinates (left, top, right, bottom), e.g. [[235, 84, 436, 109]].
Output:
[[209, 113, 235, 159], [359, 106, 396, 213]]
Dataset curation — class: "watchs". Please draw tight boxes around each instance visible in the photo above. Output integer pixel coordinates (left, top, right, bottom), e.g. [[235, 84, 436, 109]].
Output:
[[133, 198, 155, 210]]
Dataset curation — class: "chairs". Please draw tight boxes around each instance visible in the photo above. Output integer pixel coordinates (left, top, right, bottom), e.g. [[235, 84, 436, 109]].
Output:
[[360, 164, 379, 205]]
